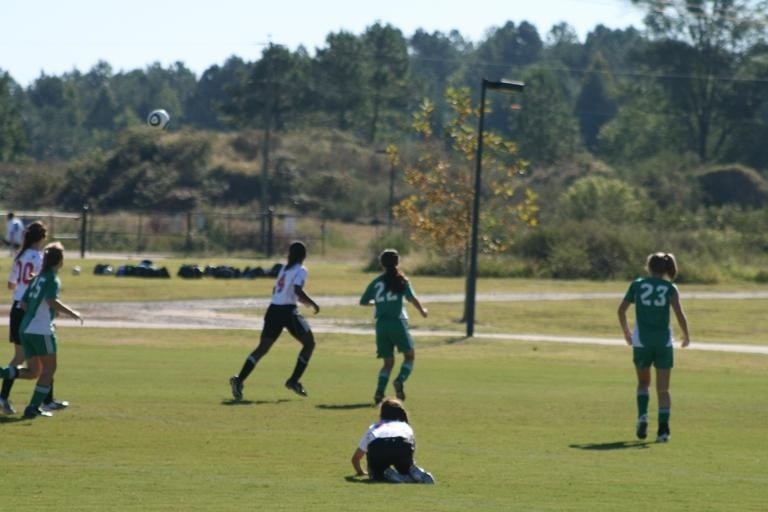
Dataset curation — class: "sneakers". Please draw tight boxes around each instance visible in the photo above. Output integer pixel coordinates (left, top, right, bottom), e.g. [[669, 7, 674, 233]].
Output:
[[375, 395, 384, 404], [40, 399, 70, 410], [285, 378, 308, 397], [657, 426, 670, 442], [0, 397, 16, 415], [23, 406, 53, 417], [384, 469, 411, 483], [229, 376, 243, 400], [408, 465, 435, 483], [393, 377, 405, 400], [636, 415, 648, 439]]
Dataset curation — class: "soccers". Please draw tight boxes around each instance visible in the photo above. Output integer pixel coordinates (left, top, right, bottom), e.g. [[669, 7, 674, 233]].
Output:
[[71, 266, 80, 275], [148, 109, 169, 130], [102, 267, 113, 275]]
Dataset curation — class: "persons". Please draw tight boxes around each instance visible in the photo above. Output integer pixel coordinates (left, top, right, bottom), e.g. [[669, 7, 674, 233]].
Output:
[[352, 397, 433, 483], [226, 240, 323, 400], [359, 247, 430, 405], [0, 221, 53, 412], [5, 212, 24, 252], [0, 240, 83, 421], [616, 251, 691, 443]]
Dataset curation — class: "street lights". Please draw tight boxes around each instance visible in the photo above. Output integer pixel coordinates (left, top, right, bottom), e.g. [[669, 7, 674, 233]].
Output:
[[464, 74, 524, 337]]
